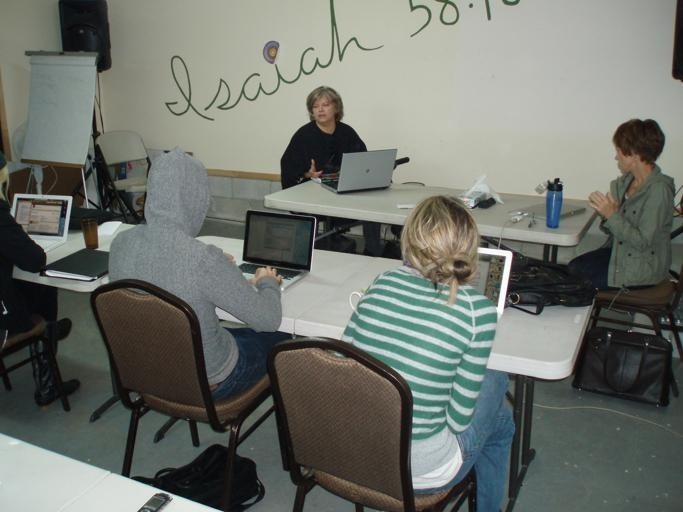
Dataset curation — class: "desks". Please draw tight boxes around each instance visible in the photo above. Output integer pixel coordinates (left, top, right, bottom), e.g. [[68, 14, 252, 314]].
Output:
[[264, 177, 600, 261], [11, 223, 594, 418]]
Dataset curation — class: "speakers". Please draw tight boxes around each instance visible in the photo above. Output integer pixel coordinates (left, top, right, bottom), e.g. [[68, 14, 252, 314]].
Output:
[[58, 0, 111, 72]]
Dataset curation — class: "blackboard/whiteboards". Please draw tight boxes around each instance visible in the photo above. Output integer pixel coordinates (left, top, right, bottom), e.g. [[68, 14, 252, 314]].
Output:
[[94, 0, 683, 217]]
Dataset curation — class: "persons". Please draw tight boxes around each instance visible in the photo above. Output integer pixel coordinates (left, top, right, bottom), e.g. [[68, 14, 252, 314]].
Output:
[[0, 196, 81, 407], [334, 193, 517, 511], [108, 150, 291, 404], [568, 118, 678, 290], [280, 85, 367, 188]]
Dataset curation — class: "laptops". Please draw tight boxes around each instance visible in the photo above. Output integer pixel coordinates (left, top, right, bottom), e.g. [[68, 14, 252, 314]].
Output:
[[318, 148, 398, 194], [463, 247, 514, 322], [524, 201, 587, 222], [236, 208, 318, 295], [9, 192, 74, 259]]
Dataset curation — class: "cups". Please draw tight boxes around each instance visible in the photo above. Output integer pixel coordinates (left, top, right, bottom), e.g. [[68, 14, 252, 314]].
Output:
[[349, 288, 366, 312], [80, 218, 98, 250]]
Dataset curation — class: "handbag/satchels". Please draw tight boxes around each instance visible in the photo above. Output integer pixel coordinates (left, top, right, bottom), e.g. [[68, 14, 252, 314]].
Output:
[[130, 443, 266, 512], [571, 326, 674, 408], [503, 263, 598, 317]]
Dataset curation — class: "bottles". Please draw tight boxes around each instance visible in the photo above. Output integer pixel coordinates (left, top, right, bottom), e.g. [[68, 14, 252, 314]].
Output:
[[545, 178, 563, 229]]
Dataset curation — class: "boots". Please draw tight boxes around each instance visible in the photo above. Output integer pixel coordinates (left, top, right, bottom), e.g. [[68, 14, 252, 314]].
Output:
[[26, 336, 81, 407], [57, 317, 73, 341]]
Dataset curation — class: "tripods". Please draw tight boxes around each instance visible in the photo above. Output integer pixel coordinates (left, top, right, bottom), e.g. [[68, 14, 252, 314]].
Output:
[[68, 94, 140, 224]]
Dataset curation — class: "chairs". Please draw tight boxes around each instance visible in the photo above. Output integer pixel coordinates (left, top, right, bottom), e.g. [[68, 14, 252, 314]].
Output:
[[94, 129, 150, 222], [266, 336, 476, 508], [90, 278, 289, 508], [573, 277, 681, 402], [1, 313, 72, 413]]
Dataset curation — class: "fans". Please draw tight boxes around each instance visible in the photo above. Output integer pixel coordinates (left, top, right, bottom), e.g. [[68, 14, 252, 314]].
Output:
[[12, 121, 49, 194]]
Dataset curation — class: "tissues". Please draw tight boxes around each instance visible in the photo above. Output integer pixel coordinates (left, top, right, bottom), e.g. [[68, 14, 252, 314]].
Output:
[[455, 184, 504, 209]]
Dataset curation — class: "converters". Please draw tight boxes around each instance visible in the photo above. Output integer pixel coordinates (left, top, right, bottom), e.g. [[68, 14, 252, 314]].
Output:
[[510, 209, 528, 223]]
